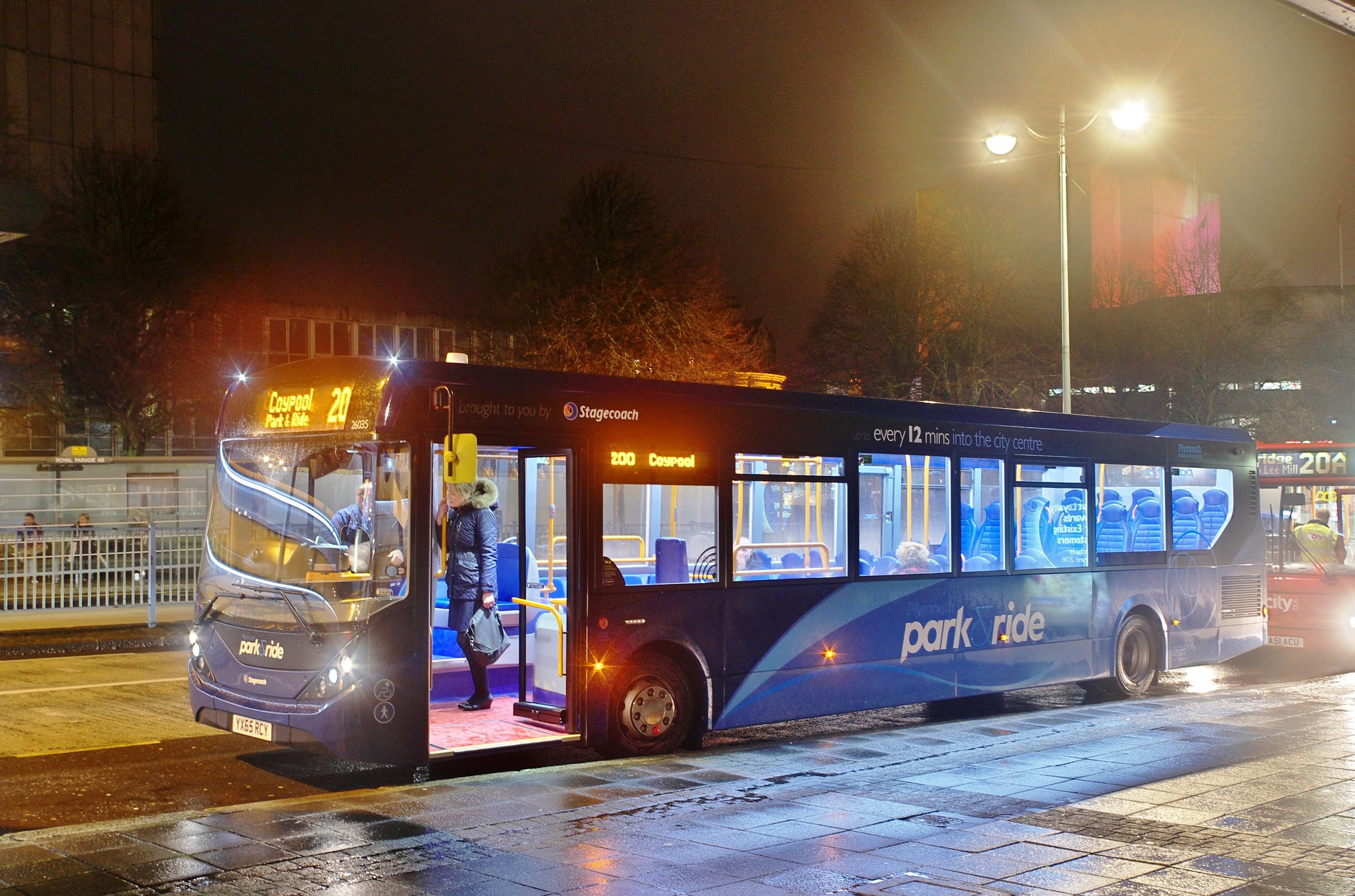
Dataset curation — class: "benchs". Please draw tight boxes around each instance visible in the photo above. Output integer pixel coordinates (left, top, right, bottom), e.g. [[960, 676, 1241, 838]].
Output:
[[430, 541, 540, 629], [0, 532, 203, 579]]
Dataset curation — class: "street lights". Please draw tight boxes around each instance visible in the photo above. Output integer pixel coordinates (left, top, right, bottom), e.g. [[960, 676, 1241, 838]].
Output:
[[982, 85, 1154, 413]]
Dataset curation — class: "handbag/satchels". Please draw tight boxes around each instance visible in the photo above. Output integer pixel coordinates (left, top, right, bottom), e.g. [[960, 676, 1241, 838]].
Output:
[[464, 602, 511, 665]]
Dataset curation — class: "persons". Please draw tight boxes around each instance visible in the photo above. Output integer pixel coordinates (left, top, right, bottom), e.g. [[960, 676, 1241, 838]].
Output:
[[17, 513, 44, 583], [69, 513, 95, 583], [128, 509, 148, 581], [436, 474, 498, 711], [319, 482, 404, 578], [1287, 508, 1347, 564], [732, 537, 764, 570], [890, 542, 931, 574]]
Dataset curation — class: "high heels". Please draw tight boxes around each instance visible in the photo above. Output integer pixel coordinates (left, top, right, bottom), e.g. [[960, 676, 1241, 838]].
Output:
[[458, 694, 493, 711]]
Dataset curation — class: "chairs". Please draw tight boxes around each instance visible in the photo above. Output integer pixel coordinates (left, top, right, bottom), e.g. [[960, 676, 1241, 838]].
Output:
[[756, 551, 774, 580], [777, 554, 807, 579], [536, 577, 567, 621], [883, 550, 898, 560], [924, 487, 1229, 571], [832, 558, 872, 578], [872, 555, 901, 575], [859, 549, 875, 567], [802, 550, 826, 578], [622, 574, 643, 585], [647, 573, 657, 584]]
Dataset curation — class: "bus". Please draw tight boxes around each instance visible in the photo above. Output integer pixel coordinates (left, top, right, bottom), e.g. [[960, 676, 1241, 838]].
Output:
[[186, 352, 1271, 768], [1251, 440, 1354, 672]]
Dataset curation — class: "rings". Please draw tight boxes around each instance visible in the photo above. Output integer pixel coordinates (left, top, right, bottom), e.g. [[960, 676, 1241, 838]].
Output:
[[490, 604, 492, 606]]
